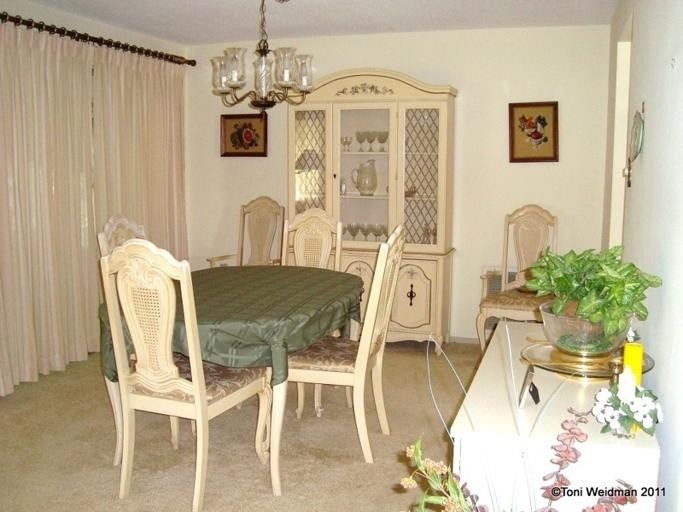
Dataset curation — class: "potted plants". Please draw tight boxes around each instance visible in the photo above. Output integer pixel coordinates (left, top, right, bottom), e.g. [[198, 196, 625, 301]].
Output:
[[518, 242, 663, 358]]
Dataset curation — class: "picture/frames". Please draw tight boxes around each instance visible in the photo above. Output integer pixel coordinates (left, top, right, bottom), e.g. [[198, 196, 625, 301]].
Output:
[[508, 101, 559, 163], [220, 114, 268, 157]]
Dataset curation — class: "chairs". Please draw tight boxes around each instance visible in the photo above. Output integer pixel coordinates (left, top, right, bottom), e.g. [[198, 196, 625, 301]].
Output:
[[475, 205, 558, 353]]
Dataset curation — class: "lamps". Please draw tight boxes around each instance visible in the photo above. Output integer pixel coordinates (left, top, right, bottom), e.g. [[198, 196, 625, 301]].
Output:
[[209, 1, 313, 120]]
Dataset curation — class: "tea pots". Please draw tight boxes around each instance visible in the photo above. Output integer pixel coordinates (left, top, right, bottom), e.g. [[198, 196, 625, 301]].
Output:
[[351, 159, 377, 196]]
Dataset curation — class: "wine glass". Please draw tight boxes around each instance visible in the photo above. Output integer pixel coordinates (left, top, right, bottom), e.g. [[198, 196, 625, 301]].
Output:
[[341, 136, 352, 152], [343, 223, 388, 242], [355, 131, 389, 152]]
